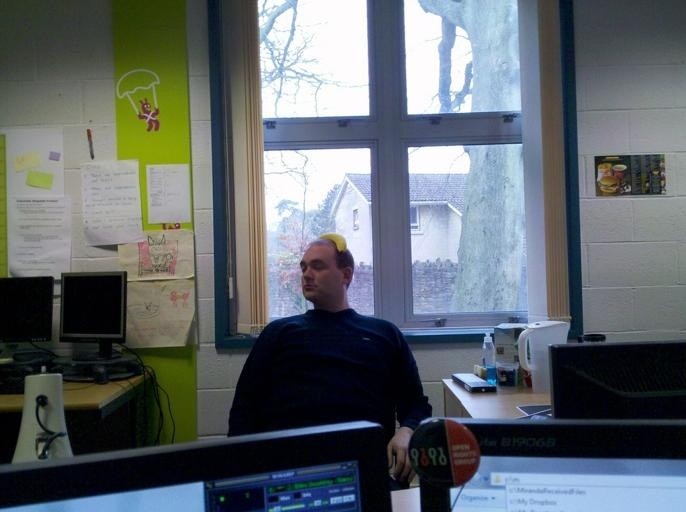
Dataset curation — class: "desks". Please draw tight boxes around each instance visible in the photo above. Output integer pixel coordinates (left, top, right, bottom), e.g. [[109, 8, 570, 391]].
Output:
[[0, 363, 155, 470], [441, 370, 553, 424], [391, 451, 686, 511]]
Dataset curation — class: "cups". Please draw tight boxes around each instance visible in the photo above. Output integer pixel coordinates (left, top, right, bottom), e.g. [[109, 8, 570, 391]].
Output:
[[495, 361, 521, 393], [612, 165, 628, 180]]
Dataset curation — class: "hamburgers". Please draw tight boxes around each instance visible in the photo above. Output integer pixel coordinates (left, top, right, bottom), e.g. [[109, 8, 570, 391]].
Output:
[[600, 176, 618, 194]]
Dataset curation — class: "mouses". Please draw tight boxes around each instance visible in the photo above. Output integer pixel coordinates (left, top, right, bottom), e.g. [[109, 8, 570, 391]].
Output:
[[83, 366, 108, 377]]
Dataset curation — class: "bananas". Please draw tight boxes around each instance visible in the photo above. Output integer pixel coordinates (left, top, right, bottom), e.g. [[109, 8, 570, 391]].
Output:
[[318, 233, 346, 254]]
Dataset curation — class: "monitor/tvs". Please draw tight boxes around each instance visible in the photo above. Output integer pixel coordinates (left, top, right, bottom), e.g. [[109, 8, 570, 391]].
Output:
[[0, 275, 55, 362], [420, 415, 686, 512], [0, 419, 394, 512], [547, 338, 686, 418], [59, 270, 128, 361]]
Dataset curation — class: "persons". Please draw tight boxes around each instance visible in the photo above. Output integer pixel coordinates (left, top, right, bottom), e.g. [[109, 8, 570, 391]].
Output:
[[226, 239, 433, 512]]
